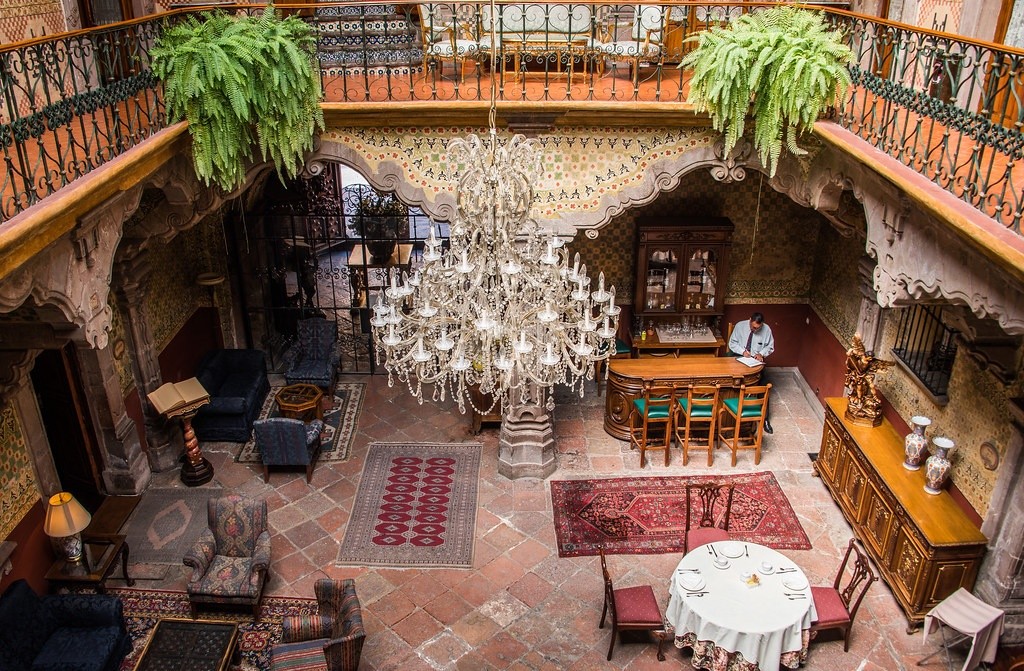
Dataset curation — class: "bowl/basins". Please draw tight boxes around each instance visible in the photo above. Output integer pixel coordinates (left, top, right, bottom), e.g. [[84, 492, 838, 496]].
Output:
[[717, 556, 728, 566], [761, 561, 772, 571]]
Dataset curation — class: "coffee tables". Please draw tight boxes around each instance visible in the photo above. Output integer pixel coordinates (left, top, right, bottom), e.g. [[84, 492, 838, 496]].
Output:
[[132, 617, 242, 671], [503, 38, 588, 85], [275, 384, 323, 421]]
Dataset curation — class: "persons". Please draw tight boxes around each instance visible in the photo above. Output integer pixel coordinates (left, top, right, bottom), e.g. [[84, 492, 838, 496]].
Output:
[[726, 312, 774, 433]]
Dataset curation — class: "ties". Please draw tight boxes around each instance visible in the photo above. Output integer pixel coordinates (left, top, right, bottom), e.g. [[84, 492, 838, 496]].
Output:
[[745, 330, 753, 352]]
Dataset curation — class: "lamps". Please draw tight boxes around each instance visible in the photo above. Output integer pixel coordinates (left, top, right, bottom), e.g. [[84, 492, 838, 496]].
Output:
[[195, 273, 225, 308], [370, 1, 620, 418], [43, 492, 92, 562]]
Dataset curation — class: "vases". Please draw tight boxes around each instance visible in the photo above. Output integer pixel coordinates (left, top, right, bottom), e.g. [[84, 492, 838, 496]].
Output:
[[366, 239, 395, 258], [923, 437, 954, 495], [902, 416, 931, 471]]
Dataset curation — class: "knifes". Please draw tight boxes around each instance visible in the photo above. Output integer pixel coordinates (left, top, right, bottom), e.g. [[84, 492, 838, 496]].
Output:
[[687, 592, 710, 594], [776, 570, 797, 574], [711, 544, 717, 558]]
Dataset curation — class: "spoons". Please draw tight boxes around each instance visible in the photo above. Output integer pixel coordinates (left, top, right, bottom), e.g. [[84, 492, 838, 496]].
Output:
[[780, 567, 794, 570], [687, 594, 703, 597]]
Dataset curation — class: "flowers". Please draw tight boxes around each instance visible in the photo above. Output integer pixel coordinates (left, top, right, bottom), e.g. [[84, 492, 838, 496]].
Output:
[[347, 196, 408, 239]]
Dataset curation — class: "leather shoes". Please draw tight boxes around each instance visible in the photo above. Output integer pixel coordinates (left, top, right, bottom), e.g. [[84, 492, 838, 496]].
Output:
[[763, 420, 773, 434]]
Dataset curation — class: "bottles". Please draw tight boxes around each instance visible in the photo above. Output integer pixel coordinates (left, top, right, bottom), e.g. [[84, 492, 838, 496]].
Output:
[[902, 415, 932, 471], [647, 292, 712, 311], [923, 436, 954, 495], [634, 316, 654, 340]]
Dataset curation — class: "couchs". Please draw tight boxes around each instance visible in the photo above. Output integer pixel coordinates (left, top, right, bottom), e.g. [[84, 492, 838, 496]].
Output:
[[191, 349, 271, 443], [0, 578, 134, 671]]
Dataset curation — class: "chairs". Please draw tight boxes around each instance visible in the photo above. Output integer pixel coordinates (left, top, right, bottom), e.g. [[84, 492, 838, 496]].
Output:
[[629, 385, 677, 468], [594, 312, 631, 396], [810, 538, 879, 653], [682, 482, 736, 558], [674, 384, 721, 467], [599, 544, 666, 661], [687, 268, 704, 292], [417, 2, 489, 86], [726, 322, 736, 357], [647, 268, 668, 293], [718, 384, 773, 467], [183, 497, 271, 622], [599, 4, 671, 82], [254, 416, 325, 484], [281, 318, 341, 397], [270, 579, 366, 671]]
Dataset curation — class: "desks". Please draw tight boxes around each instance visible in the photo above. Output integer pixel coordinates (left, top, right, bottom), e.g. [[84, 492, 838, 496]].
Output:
[[628, 327, 726, 359], [663, 541, 818, 671], [343, 244, 414, 315], [45, 533, 135, 595], [177, 410, 215, 486], [647, 292, 715, 308]]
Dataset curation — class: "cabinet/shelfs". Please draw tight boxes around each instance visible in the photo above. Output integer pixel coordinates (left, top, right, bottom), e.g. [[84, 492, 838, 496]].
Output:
[[633, 216, 735, 331], [604, 357, 765, 443], [812, 397, 990, 634], [468, 383, 510, 431], [271, 161, 348, 255]]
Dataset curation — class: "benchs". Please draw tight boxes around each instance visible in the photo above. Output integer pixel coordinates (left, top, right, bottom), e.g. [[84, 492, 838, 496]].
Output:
[[478, 4, 604, 74]]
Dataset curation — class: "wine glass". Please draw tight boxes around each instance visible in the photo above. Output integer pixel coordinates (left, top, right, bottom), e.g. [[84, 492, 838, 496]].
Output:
[[659, 315, 708, 339]]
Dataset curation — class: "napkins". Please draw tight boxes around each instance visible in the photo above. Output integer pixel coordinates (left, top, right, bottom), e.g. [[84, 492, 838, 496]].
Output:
[[683, 573, 702, 587]]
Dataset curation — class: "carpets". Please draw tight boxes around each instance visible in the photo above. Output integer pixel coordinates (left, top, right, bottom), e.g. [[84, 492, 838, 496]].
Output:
[[116, 489, 224, 566], [235, 382, 367, 463], [333, 442, 485, 569], [551, 472, 814, 557], [105, 588, 317, 671]]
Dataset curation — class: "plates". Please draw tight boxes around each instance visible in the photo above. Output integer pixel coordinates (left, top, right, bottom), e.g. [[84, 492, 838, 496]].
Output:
[[782, 573, 808, 591], [679, 574, 706, 591], [757, 567, 775, 575], [718, 542, 744, 558], [713, 561, 730, 569]]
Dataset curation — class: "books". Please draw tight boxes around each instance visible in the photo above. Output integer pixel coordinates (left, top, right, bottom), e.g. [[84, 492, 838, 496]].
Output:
[[145, 376, 211, 417]]
